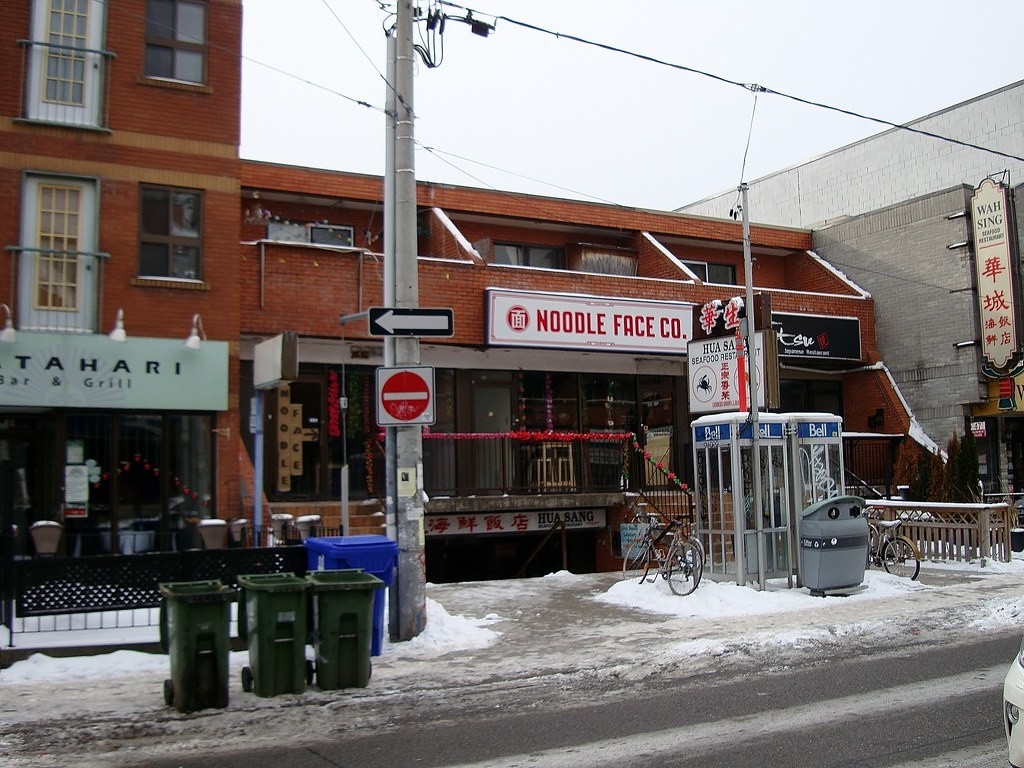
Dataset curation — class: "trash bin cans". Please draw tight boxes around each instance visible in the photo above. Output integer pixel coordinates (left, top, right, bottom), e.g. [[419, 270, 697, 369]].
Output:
[[801, 495, 870, 598], [159, 578, 239, 714], [237, 572, 311, 698], [305, 535, 401, 656], [305, 568, 385, 690]]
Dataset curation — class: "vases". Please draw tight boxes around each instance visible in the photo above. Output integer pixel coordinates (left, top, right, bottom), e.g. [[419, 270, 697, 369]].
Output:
[[197, 522, 226, 550], [296, 514, 323, 542], [30, 520, 62, 555], [231, 520, 251, 545], [269, 514, 293, 543]]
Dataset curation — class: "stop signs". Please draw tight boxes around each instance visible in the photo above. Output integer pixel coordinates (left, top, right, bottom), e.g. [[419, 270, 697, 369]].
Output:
[[376, 365, 436, 427]]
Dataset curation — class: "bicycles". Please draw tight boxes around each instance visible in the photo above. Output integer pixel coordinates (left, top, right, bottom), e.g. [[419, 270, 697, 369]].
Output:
[[861, 506, 921, 579], [622, 513, 705, 596]]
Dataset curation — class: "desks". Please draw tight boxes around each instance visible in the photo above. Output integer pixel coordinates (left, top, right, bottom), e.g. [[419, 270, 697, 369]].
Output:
[[522, 439, 576, 492]]
